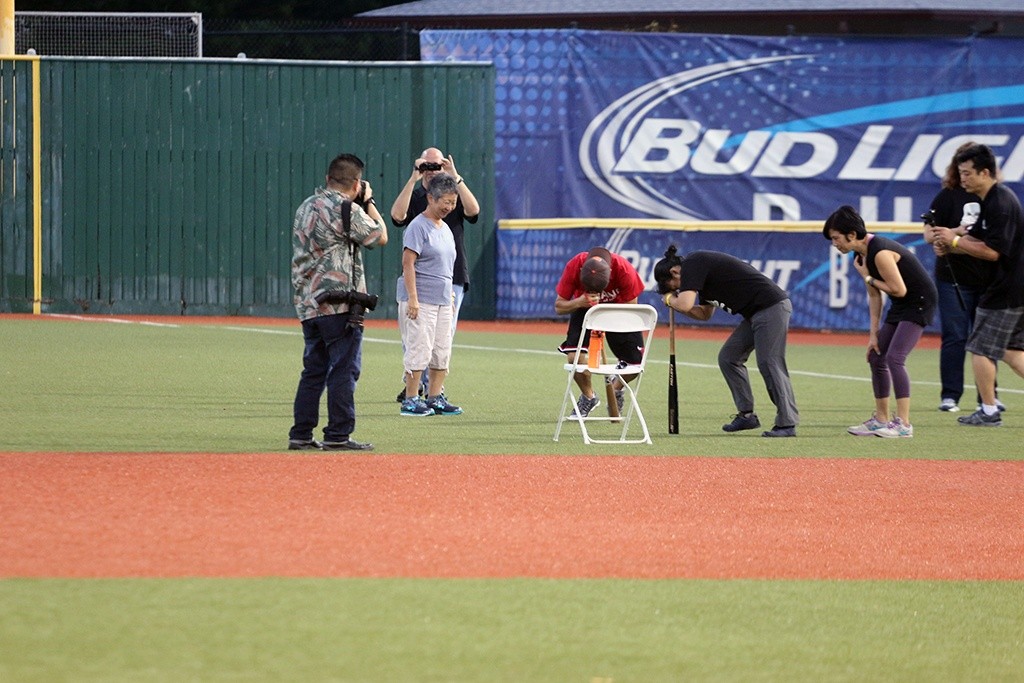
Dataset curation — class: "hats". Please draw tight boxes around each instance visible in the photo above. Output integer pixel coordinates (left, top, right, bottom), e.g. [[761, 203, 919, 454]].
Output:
[[579, 247, 613, 290]]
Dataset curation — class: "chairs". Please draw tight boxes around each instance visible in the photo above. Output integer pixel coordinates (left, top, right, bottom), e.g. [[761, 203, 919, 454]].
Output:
[[554, 304, 658, 444]]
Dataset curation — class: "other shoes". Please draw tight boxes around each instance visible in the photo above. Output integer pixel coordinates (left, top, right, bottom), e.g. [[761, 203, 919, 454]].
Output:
[[396, 385, 423, 402], [288, 437, 322, 451], [321, 438, 374, 452], [425, 393, 447, 401], [761, 425, 797, 437], [977, 399, 1005, 411], [938, 403, 960, 412], [722, 413, 761, 432]]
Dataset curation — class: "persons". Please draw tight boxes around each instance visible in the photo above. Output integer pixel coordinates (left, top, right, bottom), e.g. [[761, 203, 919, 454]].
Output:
[[654, 244, 800, 438], [287, 154, 387, 452], [931, 145, 1024, 428], [554, 246, 645, 423], [822, 205, 938, 437], [922, 141, 1006, 413], [390, 148, 480, 405], [400, 173, 465, 417]]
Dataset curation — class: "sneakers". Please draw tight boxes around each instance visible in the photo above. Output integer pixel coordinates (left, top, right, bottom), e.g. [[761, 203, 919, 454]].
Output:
[[957, 409, 1002, 427], [847, 410, 890, 436], [608, 375, 625, 418], [873, 411, 912, 438], [428, 395, 463, 416], [400, 396, 435, 416], [569, 391, 601, 422]]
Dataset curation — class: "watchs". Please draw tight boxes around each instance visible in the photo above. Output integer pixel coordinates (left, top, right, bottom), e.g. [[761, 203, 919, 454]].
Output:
[[869, 278, 874, 287]]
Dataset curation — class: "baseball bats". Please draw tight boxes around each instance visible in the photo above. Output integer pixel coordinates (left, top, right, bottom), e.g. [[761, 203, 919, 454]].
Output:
[[668, 305, 680, 435], [590, 290, 621, 425]]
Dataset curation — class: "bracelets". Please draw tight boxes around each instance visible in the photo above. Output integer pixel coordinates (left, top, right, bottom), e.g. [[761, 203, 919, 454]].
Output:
[[456, 176, 464, 184], [666, 294, 672, 306], [952, 236, 960, 247], [864, 276, 872, 283]]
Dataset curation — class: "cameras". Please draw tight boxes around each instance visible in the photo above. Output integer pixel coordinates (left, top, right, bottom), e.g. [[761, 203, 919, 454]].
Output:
[[419, 161, 442, 174], [353, 182, 366, 206], [316, 290, 378, 335]]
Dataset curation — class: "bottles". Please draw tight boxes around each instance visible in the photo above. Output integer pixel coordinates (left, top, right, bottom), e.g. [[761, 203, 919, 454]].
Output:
[[588, 330, 601, 368]]
